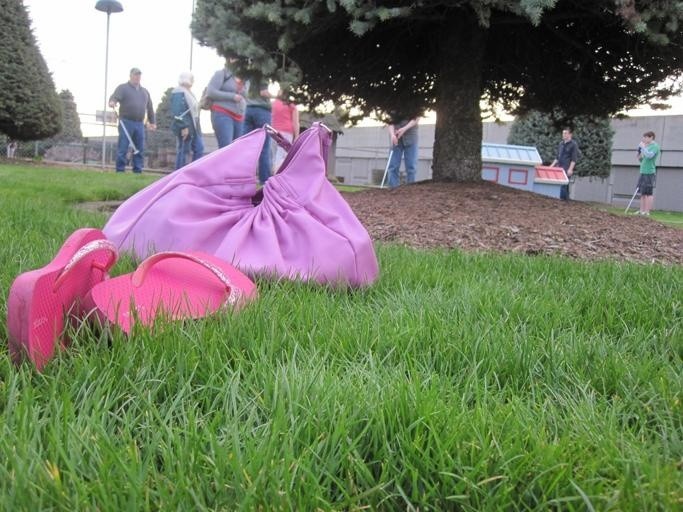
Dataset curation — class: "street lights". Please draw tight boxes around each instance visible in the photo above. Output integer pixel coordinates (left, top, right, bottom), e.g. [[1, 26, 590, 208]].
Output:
[[94, 1, 124, 167]]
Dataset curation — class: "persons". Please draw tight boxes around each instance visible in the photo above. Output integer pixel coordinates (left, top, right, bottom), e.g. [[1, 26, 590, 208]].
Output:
[[7, 136, 17, 158], [635, 131, 660, 215], [549, 126, 578, 199], [205, 57, 248, 148], [171, 72, 204, 169], [270, 89, 300, 177], [386, 116, 419, 186], [108, 68, 157, 175], [242, 77, 279, 185]]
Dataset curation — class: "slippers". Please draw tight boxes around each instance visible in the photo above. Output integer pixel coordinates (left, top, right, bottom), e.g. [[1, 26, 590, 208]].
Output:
[[5, 227, 259, 374]]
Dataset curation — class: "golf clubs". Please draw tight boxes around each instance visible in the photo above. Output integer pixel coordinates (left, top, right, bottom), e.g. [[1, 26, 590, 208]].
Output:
[[380, 145, 394, 189], [174, 101, 201, 120], [114, 106, 139, 155]]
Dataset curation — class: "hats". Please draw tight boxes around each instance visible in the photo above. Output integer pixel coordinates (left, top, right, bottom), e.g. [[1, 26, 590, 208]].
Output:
[[130, 66, 142, 74]]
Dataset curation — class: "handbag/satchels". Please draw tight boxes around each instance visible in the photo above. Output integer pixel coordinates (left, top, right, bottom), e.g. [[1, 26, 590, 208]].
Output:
[[199, 86, 212, 110], [101, 119, 380, 289]]
